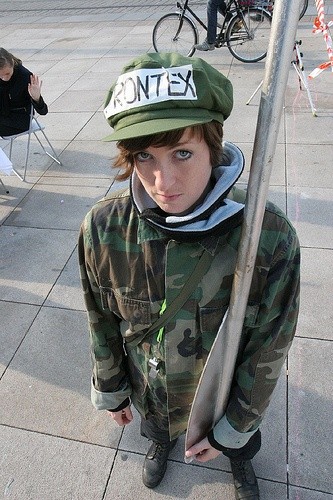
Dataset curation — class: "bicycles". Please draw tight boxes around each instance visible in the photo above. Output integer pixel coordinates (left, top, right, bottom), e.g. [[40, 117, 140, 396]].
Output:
[[152, 0, 308, 67]]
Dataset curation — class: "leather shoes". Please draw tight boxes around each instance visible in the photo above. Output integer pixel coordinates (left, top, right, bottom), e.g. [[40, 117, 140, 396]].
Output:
[[229, 459, 259, 500], [142, 437, 178, 488]]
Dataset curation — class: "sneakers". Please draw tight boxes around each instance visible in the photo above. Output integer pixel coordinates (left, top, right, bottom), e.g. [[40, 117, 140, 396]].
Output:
[[194, 41, 215, 51]]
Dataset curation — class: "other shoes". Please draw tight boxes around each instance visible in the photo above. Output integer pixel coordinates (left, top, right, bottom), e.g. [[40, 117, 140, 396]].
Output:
[[250, 14, 264, 22]]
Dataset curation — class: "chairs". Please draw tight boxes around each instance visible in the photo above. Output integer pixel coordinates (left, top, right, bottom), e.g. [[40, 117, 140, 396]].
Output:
[[1, 103, 63, 182]]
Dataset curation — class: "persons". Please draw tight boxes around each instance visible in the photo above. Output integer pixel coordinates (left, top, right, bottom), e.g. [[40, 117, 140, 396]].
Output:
[[79, 51, 301, 500], [0, 47, 48, 137], [249, 14, 264, 22], [193, 0, 241, 51]]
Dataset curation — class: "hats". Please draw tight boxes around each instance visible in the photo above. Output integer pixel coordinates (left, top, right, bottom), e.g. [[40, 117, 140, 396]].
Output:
[[101, 52, 234, 143]]
